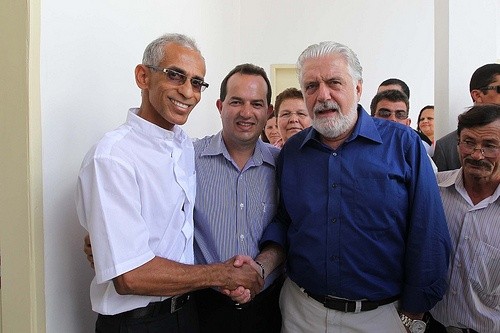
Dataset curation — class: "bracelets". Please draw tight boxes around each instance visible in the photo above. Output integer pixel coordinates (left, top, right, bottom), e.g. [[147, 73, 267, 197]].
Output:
[[256, 261, 265, 279]]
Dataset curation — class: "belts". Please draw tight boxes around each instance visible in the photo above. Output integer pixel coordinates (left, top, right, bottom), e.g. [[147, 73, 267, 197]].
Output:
[[292, 279, 403, 313], [119, 293, 199, 320]]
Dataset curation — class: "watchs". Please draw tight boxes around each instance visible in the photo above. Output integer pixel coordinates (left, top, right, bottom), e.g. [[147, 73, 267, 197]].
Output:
[[399, 312, 429, 333]]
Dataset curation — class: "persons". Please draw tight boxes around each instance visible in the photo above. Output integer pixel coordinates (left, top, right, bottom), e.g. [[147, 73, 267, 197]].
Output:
[[260, 64, 500, 173], [218, 42, 453, 333], [76, 33, 265, 333], [423, 104, 500, 333], [83, 64, 282, 333]]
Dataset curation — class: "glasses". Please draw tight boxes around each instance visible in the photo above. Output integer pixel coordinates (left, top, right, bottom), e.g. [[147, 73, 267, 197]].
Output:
[[458, 143, 499, 157], [146, 64, 209, 92], [373, 109, 408, 121], [481, 85, 500, 95]]
